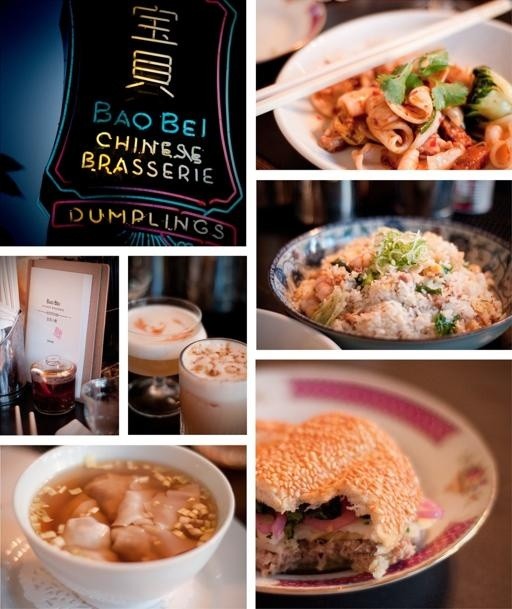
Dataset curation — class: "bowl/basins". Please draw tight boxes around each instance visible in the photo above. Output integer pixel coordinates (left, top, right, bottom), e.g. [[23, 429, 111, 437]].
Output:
[[11, 447, 237, 609]]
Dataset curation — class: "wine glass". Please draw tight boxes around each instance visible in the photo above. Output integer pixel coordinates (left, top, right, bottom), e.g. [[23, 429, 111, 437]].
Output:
[[129, 293, 210, 421]]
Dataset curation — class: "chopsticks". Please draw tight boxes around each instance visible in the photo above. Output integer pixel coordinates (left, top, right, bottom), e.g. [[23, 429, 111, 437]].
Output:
[[255, 1, 512, 122]]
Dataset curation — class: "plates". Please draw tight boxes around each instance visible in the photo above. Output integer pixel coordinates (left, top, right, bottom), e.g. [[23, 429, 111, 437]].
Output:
[[255, 1, 332, 65], [266, 211, 511, 349], [273, 9, 512, 169], [252, 364, 496, 596]]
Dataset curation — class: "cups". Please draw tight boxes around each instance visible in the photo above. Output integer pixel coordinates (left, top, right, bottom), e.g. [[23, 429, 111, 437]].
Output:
[[29, 363, 120, 439], [175, 335, 248, 435]]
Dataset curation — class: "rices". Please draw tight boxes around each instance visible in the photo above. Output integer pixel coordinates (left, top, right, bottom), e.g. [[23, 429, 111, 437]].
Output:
[[288, 226, 507, 340]]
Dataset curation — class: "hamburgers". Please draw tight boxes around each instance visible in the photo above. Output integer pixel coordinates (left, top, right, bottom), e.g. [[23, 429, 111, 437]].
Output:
[[256, 412, 443, 581]]
